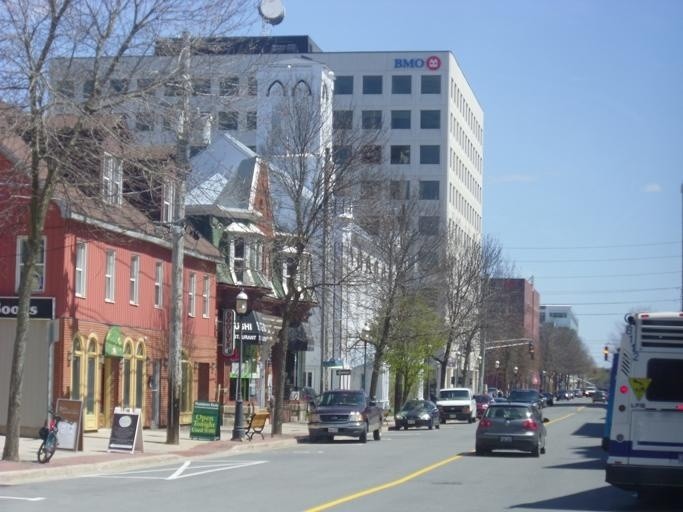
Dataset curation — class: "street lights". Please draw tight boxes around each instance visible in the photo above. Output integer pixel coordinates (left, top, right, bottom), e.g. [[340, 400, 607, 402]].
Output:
[[318, 212, 354, 394], [232, 286, 249, 440], [358, 323, 548, 382]]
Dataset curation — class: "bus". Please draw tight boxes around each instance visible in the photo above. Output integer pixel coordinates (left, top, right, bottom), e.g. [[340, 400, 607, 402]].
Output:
[[602, 310, 683, 486]]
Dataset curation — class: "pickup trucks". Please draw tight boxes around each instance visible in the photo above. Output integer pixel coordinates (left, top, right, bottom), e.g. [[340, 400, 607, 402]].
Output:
[[305, 390, 384, 447]]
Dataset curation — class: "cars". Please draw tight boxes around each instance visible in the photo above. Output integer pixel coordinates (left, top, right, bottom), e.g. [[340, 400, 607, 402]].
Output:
[[394, 399, 441, 430], [474, 385, 608, 420], [474, 402, 550, 456]]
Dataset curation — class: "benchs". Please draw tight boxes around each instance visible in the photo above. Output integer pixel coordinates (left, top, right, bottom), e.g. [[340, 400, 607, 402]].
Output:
[[244, 413, 269, 441], [383, 409, 390, 425]]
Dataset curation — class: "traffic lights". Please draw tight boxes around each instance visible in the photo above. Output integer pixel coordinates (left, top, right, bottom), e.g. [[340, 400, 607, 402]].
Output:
[[603, 346, 608, 361], [529, 343, 535, 353]]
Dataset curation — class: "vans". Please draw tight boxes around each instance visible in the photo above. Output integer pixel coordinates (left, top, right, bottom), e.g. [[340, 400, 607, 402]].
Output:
[[437, 388, 477, 424]]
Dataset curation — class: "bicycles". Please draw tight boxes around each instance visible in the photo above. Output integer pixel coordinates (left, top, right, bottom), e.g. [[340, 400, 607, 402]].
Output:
[[36, 410, 65, 465]]
